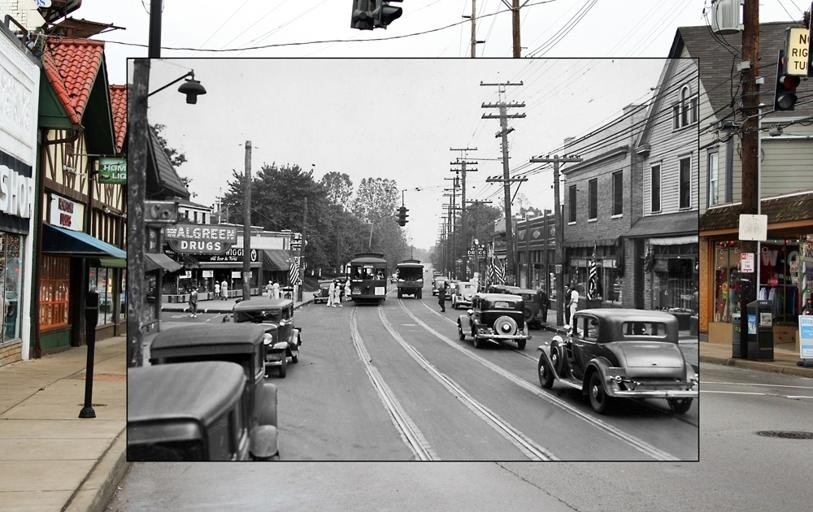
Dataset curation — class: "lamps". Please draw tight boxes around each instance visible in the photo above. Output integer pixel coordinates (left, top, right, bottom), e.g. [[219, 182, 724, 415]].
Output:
[[146, 66, 205, 106]]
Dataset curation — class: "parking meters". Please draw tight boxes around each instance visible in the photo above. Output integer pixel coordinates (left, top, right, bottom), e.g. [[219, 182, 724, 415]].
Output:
[[75, 292, 100, 419]]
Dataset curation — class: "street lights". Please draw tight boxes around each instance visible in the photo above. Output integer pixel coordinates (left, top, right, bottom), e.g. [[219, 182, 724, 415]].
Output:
[[127, 60, 206, 363]]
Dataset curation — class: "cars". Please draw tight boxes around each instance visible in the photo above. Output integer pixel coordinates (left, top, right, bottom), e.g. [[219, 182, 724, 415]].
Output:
[[390, 273, 396, 283], [431, 267, 545, 349], [311, 276, 350, 303], [127, 290, 302, 460], [90, 287, 124, 314], [536, 306, 698, 419]]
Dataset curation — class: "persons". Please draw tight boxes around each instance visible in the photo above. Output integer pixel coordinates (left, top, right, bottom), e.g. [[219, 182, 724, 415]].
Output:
[[188, 286, 197, 318], [566, 285, 578, 326], [436, 283, 446, 313], [266, 280, 273, 297], [213, 280, 219, 299], [563, 283, 571, 323], [220, 279, 228, 300], [273, 280, 280, 299], [538, 286, 550, 323], [326, 270, 384, 308]]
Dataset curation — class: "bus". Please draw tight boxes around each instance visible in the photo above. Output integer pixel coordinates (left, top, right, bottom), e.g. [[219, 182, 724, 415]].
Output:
[[395, 258, 424, 299], [349, 252, 388, 306]]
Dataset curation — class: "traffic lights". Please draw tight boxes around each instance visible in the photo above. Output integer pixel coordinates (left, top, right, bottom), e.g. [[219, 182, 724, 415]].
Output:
[[771, 49, 799, 112], [395, 207, 409, 226]]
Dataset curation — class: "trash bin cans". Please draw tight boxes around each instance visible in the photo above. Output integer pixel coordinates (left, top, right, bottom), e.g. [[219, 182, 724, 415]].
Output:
[[731, 313, 742, 358]]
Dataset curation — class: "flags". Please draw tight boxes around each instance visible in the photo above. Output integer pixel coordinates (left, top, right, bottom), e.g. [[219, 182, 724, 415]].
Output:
[[587, 245, 597, 300], [495, 257, 505, 283], [289, 261, 299, 285], [487, 259, 494, 279]]
